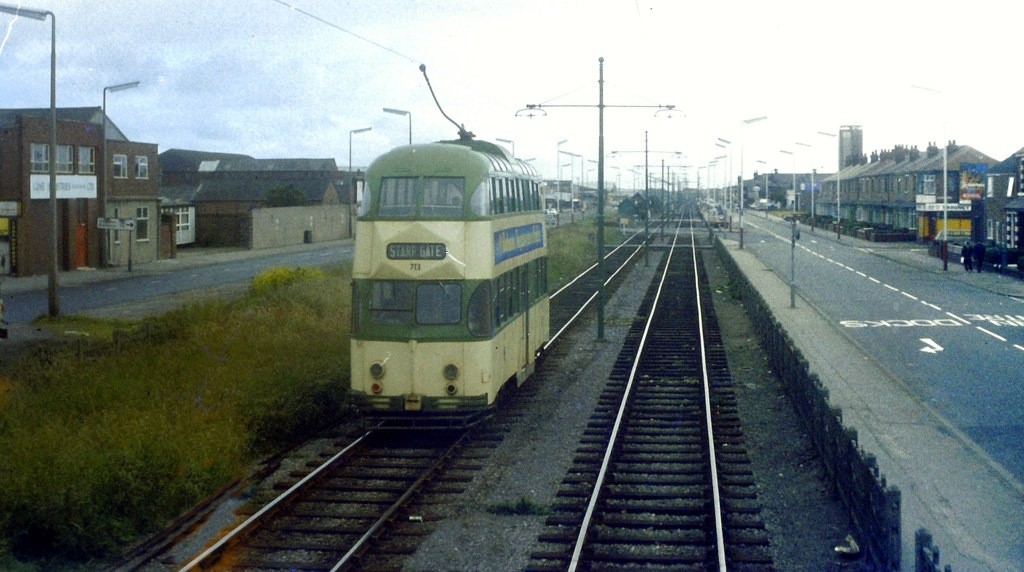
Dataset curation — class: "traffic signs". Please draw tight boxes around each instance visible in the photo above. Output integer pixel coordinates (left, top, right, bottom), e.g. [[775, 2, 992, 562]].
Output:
[[96, 216, 135, 232]]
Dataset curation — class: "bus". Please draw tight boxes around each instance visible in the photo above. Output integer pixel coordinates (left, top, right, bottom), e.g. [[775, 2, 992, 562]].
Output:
[[343, 60, 552, 430]]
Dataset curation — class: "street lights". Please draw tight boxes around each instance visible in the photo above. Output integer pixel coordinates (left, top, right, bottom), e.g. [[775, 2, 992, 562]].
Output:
[[559, 150, 576, 223], [793, 141, 815, 232], [575, 154, 584, 222], [817, 129, 842, 240], [557, 139, 569, 227], [0, 4, 60, 321], [559, 163, 570, 212], [696, 164, 706, 210], [778, 151, 797, 215], [756, 159, 769, 218], [717, 136, 733, 233], [494, 137, 515, 158], [714, 142, 727, 230], [381, 105, 413, 145], [98, 80, 141, 268], [739, 115, 770, 251], [348, 125, 373, 238]]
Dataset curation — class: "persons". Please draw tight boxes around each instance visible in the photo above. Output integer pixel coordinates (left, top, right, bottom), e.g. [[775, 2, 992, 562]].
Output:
[[796, 220, 800, 239], [961, 240, 985, 273]]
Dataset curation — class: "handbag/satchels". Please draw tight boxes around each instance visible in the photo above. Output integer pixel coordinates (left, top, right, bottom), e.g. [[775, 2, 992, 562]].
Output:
[[959, 256, 965, 264]]
